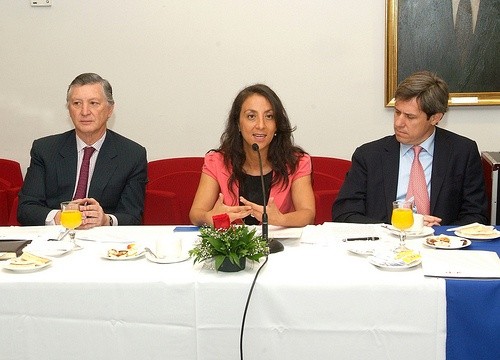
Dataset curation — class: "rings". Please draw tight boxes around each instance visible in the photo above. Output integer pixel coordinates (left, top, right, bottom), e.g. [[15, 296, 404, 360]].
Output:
[[251, 214, 254, 217]]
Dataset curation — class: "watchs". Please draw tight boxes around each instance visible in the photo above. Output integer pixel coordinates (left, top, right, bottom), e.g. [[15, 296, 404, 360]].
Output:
[[109, 216, 113, 225]]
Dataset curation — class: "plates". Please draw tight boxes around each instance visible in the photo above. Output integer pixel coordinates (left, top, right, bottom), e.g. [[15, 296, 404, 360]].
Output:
[[368, 254, 421, 269], [22, 240, 74, 257], [146, 250, 191, 263], [423, 235, 472, 249], [3, 255, 54, 273], [347, 241, 387, 254], [454, 229, 500, 239], [390, 225, 435, 236], [102, 246, 145, 260]]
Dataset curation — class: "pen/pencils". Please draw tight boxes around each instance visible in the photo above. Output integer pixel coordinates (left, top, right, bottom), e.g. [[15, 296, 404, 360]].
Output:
[[343, 237, 380, 241]]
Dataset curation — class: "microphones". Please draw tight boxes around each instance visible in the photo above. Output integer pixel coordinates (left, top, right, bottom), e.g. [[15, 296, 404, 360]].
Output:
[[252, 142, 283, 255]]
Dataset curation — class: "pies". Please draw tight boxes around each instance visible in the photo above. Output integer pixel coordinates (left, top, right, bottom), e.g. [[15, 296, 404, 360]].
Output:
[[455, 224, 497, 235]]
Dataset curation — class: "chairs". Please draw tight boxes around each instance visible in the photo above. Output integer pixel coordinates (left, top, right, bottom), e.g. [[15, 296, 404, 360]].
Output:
[[143, 157, 206, 226], [0, 158, 24, 228], [311, 156, 352, 225]]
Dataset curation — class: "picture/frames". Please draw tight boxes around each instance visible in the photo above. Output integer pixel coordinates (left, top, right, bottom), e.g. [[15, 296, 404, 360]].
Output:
[[384, 0, 500, 108]]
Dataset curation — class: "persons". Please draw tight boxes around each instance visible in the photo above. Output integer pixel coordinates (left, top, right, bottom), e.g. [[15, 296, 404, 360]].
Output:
[[332, 71, 489, 225], [188, 84, 316, 228], [16, 73, 147, 230]]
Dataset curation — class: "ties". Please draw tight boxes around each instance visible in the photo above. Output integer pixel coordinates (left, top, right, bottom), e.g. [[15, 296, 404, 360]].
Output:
[[404, 146, 430, 215], [73, 147, 95, 201]]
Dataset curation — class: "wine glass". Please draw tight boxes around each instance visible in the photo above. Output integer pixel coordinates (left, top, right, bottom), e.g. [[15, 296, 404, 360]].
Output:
[[390, 200, 414, 252], [60, 200, 83, 251]]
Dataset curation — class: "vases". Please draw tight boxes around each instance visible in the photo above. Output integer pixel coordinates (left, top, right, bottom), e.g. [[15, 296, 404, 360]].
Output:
[[216, 253, 247, 271]]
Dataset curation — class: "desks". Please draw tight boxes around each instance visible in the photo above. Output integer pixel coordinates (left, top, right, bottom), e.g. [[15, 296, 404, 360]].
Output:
[[0, 226, 500, 360]]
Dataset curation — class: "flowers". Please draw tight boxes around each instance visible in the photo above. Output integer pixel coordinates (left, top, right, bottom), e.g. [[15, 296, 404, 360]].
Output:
[[189, 215, 270, 272]]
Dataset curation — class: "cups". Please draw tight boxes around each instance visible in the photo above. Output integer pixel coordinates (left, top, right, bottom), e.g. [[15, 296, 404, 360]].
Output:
[[413, 213, 424, 235]]
